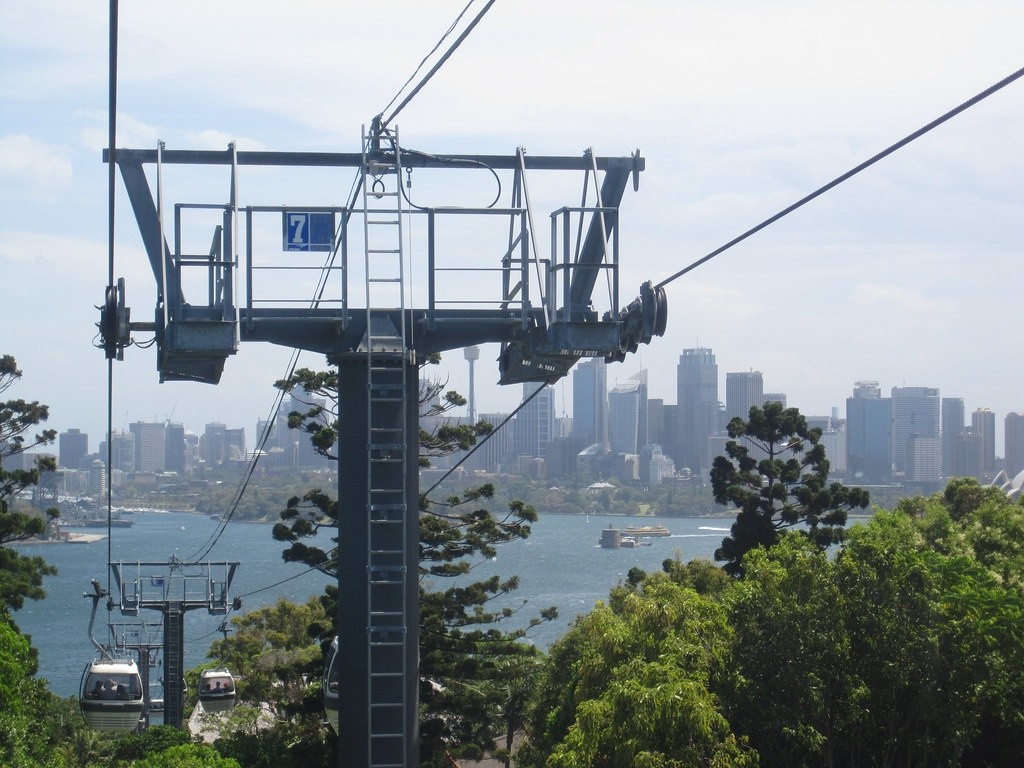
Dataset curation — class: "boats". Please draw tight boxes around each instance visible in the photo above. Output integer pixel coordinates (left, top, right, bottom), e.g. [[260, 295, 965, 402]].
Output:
[[619, 523, 672, 538]]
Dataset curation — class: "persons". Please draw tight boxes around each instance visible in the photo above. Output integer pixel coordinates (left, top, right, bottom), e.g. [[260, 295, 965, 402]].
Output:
[[88, 679, 129, 700], [204, 681, 231, 693]]
